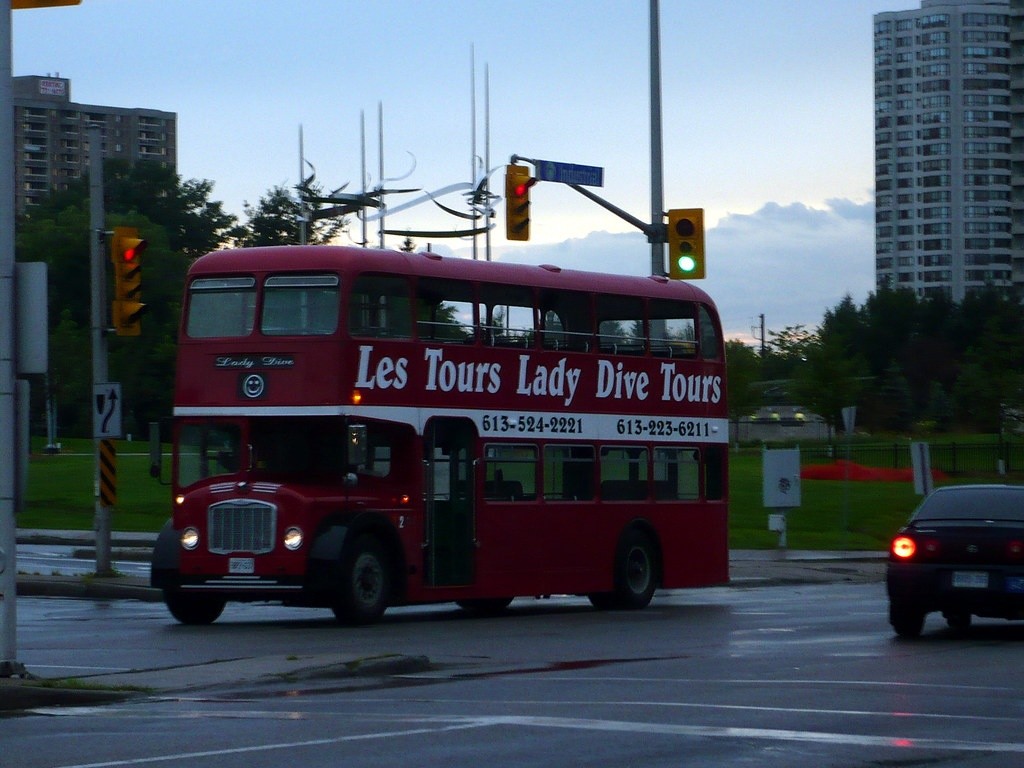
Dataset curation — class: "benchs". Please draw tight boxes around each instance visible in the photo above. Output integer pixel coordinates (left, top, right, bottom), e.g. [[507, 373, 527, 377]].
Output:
[[466, 334, 696, 359], [456, 480, 523, 502], [600, 480, 675, 501]]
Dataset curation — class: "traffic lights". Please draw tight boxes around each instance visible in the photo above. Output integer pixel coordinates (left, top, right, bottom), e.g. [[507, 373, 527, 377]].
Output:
[[505, 164, 539, 242], [669, 207, 708, 281], [110, 226, 149, 337]]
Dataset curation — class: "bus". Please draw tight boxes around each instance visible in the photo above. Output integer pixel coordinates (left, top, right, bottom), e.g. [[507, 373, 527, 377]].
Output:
[[147, 242, 735, 627]]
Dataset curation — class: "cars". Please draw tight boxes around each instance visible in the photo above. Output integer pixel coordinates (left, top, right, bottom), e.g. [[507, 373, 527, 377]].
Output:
[[886, 483, 1023, 634]]
